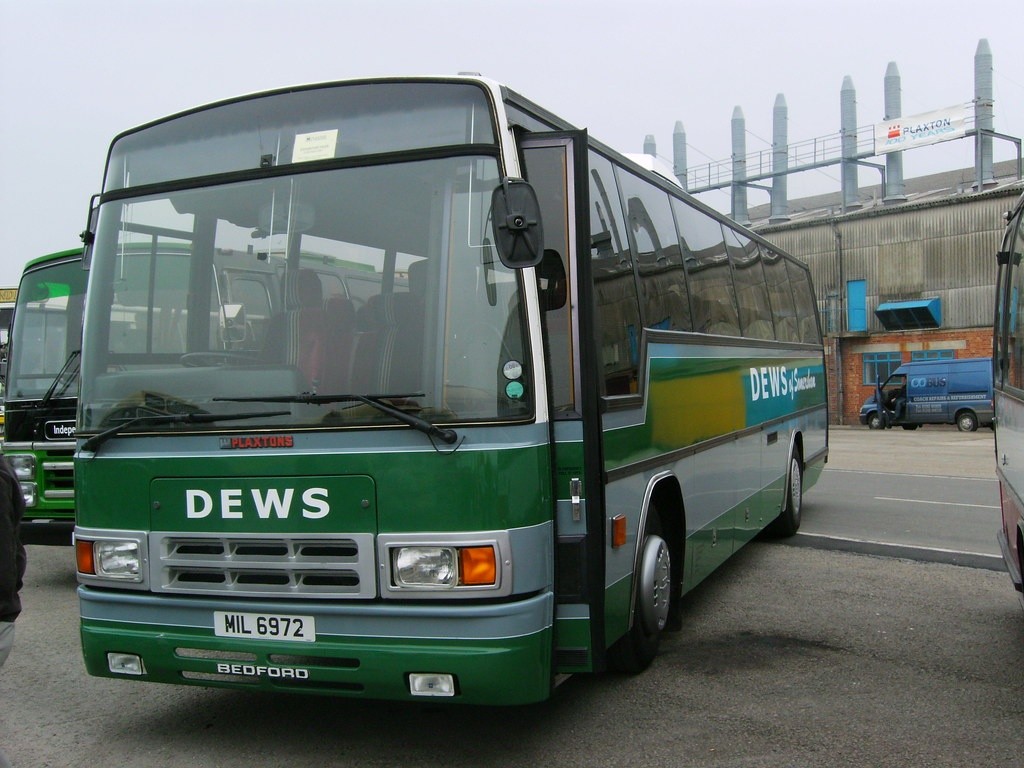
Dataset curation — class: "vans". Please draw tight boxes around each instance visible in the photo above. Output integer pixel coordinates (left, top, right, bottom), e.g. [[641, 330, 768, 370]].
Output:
[[858, 357, 994, 433], [991, 191, 1024, 587]]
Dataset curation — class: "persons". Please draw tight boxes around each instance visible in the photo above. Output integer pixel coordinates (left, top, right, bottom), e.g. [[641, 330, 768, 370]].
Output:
[[891, 376, 906, 420]]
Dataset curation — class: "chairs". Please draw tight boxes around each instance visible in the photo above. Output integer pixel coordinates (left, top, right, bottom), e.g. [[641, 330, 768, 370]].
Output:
[[262, 259, 437, 396]]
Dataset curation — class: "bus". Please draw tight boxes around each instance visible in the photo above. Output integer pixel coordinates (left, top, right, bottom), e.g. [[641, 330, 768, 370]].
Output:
[[75, 71, 827, 707], [0, 243, 427, 522]]
